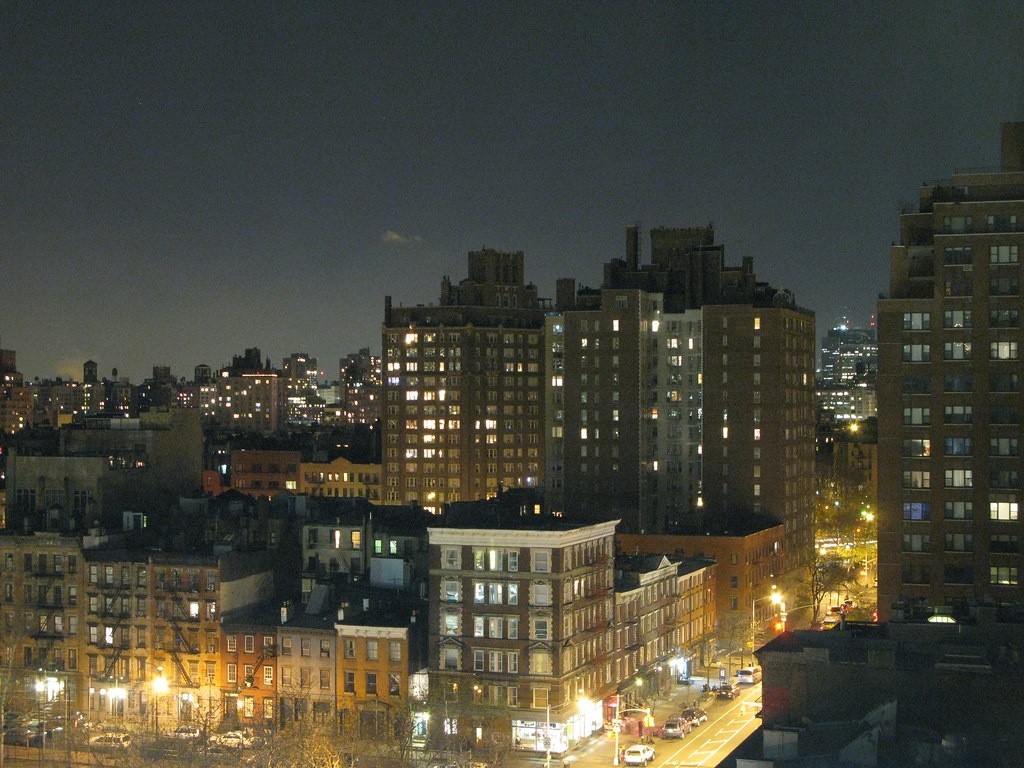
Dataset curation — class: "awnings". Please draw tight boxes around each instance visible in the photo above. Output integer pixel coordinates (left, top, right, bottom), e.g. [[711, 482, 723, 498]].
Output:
[[677, 650, 696, 662]]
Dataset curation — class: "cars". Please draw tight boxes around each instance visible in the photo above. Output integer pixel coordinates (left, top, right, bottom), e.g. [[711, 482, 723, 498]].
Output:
[[719, 684, 741, 701], [823, 598, 854, 629], [84, 733, 131, 753], [5, 699, 88, 750], [207, 731, 252, 750], [623, 745, 655, 767], [164, 725, 200, 740]]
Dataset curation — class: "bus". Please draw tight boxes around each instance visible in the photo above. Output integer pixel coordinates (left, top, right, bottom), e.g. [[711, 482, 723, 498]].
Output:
[[738, 667, 762, 685]]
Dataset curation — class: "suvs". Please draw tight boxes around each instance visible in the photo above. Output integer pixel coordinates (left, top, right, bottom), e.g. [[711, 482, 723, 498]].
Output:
[[661, 717, 692, 740], [682, 707, 708, 728]]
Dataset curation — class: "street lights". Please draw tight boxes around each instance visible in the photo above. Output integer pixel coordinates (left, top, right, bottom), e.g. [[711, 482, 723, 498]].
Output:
[[34, 670, 64, 760], [753, 593, 782, 665], [148, 678, 169, 741], [613, 694, 655, 767], [545, 697, 593, 768]]
